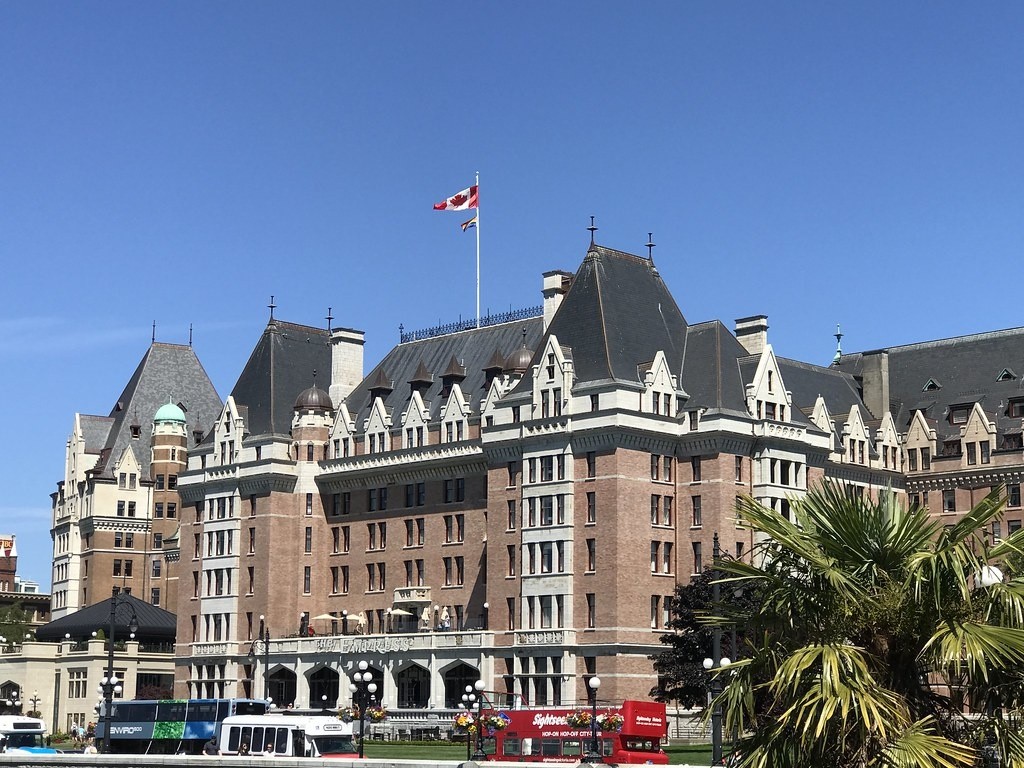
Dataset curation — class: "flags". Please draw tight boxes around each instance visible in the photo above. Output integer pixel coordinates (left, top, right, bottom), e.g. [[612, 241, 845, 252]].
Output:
[[461, 216, 477, 232], [432, 185, 479, 212]]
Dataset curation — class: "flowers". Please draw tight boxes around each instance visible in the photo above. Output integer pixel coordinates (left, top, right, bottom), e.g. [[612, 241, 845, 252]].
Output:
[[567, 711, 594, 729], [596, 712, 624, 731], [452, 712, 479, 736], [338, 707, 358, 723], [480, 711, 511, 735], [365, 704, 387, 723]]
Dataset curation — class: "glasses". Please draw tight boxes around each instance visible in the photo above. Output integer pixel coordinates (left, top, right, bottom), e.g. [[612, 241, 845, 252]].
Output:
[[243, 744, 247, 748], [266, 747, 273, 749]]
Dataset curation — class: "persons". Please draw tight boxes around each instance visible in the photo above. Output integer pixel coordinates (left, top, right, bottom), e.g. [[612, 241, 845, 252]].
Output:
[[0, 734, 6, 753], [437, 622, 445, 632], [263, 743, 276, 757], [71, 722, 97, 741], [237, 743, 251, 756], [80, 744, 92, 754], [308, 623, 315, 637], [287, 703, 292, 710], [202, 736, 222, 755], [353, 624, 360, 635]]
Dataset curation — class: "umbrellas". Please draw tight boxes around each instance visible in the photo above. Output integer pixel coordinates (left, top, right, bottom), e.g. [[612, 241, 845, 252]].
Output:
[[336, 614, 368, 633], [383, 608, 413, 634], [311, 613, 340, 635]]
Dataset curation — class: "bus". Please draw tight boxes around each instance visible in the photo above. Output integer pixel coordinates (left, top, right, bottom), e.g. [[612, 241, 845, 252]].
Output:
[[95, 697, 270, 756], [470, 690, 669, 765], [218, 716, 369, 761]]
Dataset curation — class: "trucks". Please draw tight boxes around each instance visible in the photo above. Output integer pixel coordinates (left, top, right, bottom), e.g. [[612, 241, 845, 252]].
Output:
[[0, 715, 64, 755]]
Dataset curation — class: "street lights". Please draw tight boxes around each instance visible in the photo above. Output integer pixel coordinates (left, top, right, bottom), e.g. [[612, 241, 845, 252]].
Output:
[[247, 638, 269, 699], [975, 566, 1003, 743], [472, 679, 489, 762], [5, 691, 21, 713], [349, 660, 377, 759], [103, 603, 139, 753], [457, 685, 480, 761], [583, 677, 605, 762], [322, 695, 327, 712], [29, 691, 41, 714]]
